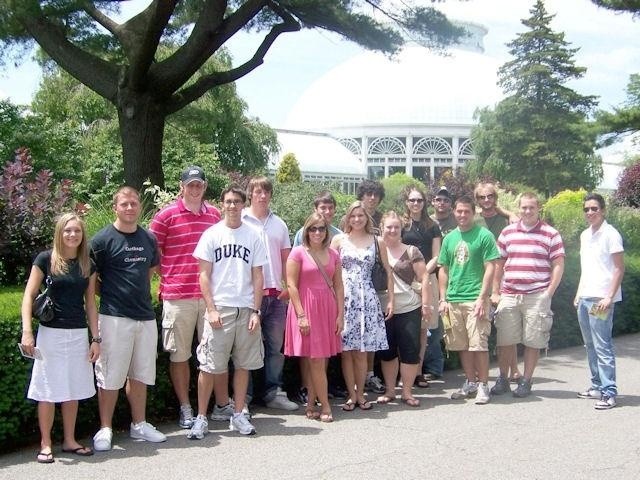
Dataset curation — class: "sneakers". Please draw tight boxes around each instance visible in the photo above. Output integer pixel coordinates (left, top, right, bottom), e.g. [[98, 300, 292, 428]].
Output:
[[266, 389, 298, 411], [180, 397, 256, 439], [337, 387, 351, 400], [365, 376, 386, 394], [93, 427, 113, 451], [297, 386, 319, 405], [577, 388, 617, 409], [130, 422, 167, 443], [451, 372, 533, 404]]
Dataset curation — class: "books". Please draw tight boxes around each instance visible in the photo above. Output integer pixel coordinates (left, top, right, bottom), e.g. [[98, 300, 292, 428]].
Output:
[[588, 304, 611, 322]]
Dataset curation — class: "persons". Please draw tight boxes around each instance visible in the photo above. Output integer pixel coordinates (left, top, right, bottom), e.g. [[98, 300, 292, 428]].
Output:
[[435, 194, 500, 405], [183, 183, 270, 440], [396, 185, 443, 389], [20, 212, 102, 464], [422, 184, 520, 381], [472, 182, 524, 383], [572, 194, 624, 410], [241, 175, 299, 414], [284, 212, 345, 423], [291, 191, 348, 404], [87, 185, 168, 452], [337, 179, 387, 395], [329, 200, 395, 411], [491, 191, 566, 397], [375, 210, 433, 406], [150, 167, 251, 428]]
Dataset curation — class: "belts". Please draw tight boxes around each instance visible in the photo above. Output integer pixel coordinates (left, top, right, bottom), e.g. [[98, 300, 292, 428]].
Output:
[[263, 289, 279, 295]]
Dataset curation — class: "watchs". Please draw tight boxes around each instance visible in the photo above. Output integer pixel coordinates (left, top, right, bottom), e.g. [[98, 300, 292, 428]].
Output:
[[253, 309, 261, 316], [90, 335, 101, 343]]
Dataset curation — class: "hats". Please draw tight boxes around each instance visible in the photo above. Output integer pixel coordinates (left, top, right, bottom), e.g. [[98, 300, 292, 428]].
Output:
[[182, 163, 206, 187], [434, 186, 455, 198]]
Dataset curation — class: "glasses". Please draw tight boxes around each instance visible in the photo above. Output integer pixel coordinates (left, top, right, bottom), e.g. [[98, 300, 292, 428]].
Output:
[[223, 200, 245, 205], [407, 198, 425, 203], [477, 193, 493, 200], [309, 227, 327, 233], [583, 207, 601, 212]]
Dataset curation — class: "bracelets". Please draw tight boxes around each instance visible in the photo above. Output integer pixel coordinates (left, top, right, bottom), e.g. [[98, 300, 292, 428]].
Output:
[[297, 312, 306, 319], [422, 304, 431, 309], [437, 299, 445, 306]]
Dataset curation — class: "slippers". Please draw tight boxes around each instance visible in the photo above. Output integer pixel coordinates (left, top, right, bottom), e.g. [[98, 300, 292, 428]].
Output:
[[304, 395, 419, 422], [37, 449, 55, 463], [414, 375, 429, 388], [62, 444, 95, 455]]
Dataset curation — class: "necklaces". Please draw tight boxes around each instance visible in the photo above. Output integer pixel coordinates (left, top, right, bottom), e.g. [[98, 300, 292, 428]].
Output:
[[481, 210, 496, 217], [412, 218, 421, 232]]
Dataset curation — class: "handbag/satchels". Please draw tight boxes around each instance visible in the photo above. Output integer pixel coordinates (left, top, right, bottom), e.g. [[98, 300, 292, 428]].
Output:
[[412, 277, 422, 294], [372, 264, 387, 291], [32, 285, 55, 322]]
